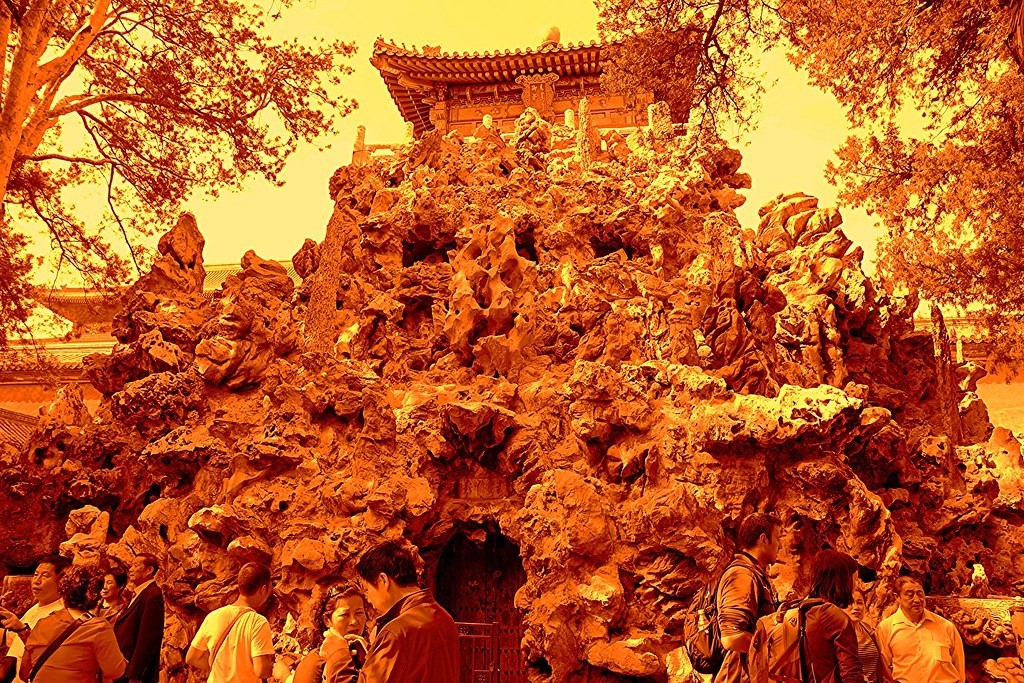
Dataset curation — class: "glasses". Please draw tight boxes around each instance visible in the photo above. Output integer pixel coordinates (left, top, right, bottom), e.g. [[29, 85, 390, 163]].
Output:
[[322, 581, 361, 610]]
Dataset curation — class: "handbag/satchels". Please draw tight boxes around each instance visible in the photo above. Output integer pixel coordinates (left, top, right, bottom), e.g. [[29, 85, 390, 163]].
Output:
[[0, 644, 17, 683]]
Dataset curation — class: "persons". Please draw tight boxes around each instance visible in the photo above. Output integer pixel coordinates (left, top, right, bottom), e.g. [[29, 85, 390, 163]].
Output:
[[19, 561, 127, 683], [94, 567, 129, 622], [709, 513, 783, 683], [116, 553, 163, 683], [0, 555, 72, 683], [292, 582, 368, 683], [876, 575, 965, 683], [185, 561, 274, 683], [842, 587, 880, 683], [321, 541, 460, 683], [749, 549, 868, 682]]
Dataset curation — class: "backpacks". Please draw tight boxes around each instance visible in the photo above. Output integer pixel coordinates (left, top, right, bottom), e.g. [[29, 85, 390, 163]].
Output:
[[684, 561, 763, 674], [749, 599, 828, 683]]
